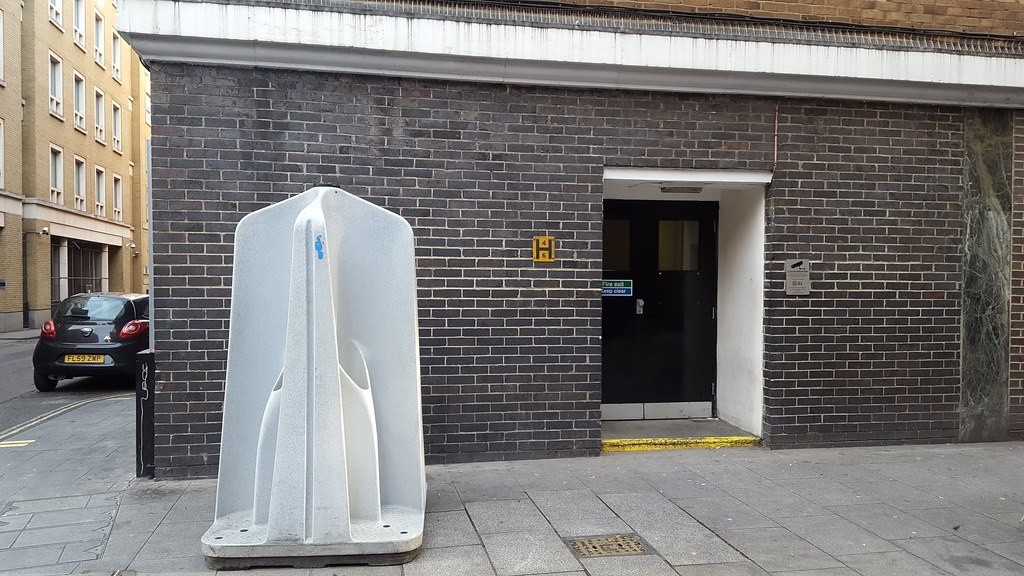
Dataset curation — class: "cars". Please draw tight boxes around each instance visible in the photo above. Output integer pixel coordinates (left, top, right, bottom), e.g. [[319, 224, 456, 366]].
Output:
[[32, 292, 149, 393]]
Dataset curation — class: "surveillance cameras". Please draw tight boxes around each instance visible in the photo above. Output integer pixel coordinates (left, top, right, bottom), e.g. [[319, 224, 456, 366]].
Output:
[[131, 243, 136, 249], [42, 227, 48, 232]]
